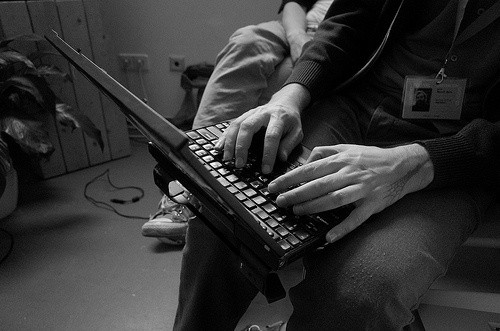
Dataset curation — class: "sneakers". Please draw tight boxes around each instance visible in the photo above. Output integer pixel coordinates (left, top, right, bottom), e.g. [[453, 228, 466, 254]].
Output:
[[141, 180, 195, 245]]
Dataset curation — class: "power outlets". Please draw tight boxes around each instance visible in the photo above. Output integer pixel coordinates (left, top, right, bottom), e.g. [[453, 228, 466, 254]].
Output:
[[119, 53, 150, 71]]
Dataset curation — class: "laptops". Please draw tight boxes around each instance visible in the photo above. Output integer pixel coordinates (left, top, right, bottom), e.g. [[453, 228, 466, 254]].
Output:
[[43, 29, 355, 270]]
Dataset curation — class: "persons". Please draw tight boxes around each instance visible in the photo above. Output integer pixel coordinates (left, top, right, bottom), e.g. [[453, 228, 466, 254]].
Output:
[[142, 0, 336, 246], [171, 0, 500, 331]]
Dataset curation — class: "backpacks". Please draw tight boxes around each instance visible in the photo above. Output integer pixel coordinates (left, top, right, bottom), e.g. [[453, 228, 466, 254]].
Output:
[[172, 64, 215, 130]]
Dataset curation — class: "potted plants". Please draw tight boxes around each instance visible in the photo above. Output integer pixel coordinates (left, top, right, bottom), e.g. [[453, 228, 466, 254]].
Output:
[[0, 22, 104, 219]]
